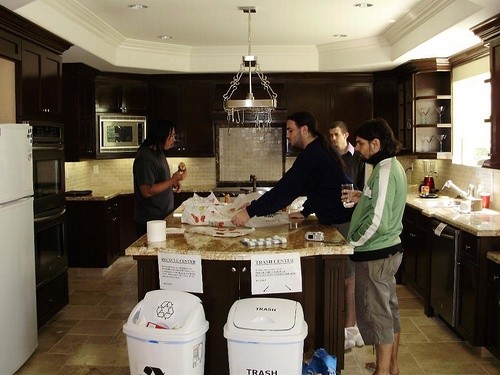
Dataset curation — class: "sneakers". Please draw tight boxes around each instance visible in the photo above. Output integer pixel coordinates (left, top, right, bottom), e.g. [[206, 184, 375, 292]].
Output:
[[344, 326, 365, 349]]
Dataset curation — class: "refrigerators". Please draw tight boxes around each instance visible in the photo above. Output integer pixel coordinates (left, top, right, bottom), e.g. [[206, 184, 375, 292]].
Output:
[[0, 122, 39, 375]]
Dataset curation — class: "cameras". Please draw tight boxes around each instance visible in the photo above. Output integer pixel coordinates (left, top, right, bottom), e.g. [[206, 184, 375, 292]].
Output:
[[305, 232, 324, 242]]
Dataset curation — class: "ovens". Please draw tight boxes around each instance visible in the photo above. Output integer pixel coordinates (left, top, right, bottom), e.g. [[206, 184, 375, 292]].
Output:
[[22, 120, 70, 329]]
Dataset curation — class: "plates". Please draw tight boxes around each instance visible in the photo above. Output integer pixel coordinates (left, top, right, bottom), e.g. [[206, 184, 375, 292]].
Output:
[[419, 193, 439, 198]]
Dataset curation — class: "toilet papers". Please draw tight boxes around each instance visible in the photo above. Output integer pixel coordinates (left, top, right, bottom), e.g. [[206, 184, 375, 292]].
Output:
[[147, 220, 166, 241]]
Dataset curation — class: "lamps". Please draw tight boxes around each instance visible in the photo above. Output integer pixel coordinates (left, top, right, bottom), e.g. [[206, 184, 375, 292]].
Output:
[[223, 6, 278, 139]]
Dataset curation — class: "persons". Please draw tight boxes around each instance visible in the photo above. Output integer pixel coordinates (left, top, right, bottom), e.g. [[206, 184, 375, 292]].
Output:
[[347, 118, 408, 375], [328, 120, 365, 193], [133, 122, 187, 240], [231, 111, 364, 351]]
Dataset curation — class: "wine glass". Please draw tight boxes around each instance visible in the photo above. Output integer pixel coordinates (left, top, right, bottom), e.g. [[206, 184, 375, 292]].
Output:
[[425, 135, 433, 152], [435, 106, 447, 124], [421, 106, 431, 124], [435, 134, 448, 152]]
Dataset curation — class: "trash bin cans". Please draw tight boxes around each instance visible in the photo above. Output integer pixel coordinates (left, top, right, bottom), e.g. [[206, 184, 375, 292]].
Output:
[[122, 289, 209, 375], [223, 297, 308, 375]]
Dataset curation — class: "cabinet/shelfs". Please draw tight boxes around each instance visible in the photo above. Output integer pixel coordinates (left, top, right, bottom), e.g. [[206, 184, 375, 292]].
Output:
[[416, 95, 452, 128], [148, 78, 214, 158], [398, 205, 431, 319], [482, 38, 500, 170], [20, 40, 64, 125], [286, 79, 398, 156], [106, 194, 185, 266], [455, 229, 486, 348]]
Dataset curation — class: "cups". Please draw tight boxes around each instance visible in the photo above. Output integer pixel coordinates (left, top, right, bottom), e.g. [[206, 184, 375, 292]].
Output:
[[289, 217, 297, 229], [424, 176, 434, 190], [472, 198, 482, 211], [421, 186, 429, 196], [341, 184, 354, 208], [479, 193, 490, 208], [147, 220, 166, 242], [460, 199, 471, 211]]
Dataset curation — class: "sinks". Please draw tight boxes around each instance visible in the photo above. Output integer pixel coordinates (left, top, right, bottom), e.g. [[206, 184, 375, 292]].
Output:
[[414, 199, 464, 208]]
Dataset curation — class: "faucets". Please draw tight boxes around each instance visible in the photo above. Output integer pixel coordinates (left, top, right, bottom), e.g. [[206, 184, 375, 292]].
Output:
[[442, 180, 474, 200]]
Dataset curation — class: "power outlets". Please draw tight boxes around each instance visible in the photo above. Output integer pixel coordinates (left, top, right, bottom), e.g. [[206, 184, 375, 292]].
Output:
[[93, 165, 99, 174], [434, 170, 439, 176], [410, 162, 413, 171]]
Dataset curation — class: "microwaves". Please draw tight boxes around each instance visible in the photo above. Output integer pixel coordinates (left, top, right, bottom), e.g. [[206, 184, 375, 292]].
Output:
[[98, 114, 147, 153]]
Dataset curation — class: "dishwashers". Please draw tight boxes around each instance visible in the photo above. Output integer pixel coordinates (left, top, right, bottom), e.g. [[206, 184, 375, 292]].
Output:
[[430, 219, 459, 329]]
